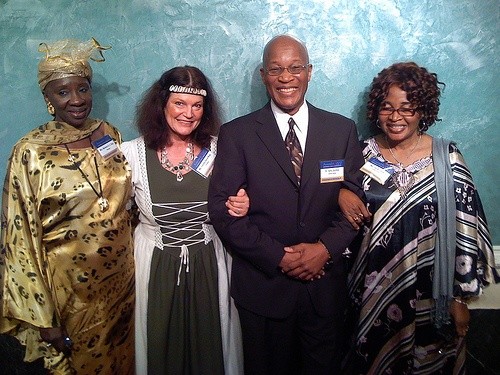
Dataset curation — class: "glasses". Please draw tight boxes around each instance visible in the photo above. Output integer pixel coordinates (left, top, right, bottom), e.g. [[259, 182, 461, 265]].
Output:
[[263, 64, 309, 75], [378, 107, 419, 116]]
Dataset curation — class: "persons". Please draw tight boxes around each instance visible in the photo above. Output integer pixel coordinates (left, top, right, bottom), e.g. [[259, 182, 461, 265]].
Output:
[[118, 64, 250, 375], [336, 62, 500, 375], [206, 34, 367, 375], [0, 52, 136, 375]]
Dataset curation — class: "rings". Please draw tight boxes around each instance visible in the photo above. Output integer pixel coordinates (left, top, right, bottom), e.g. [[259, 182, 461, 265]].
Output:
[[235, 207, 241, 214], [464, 328, 468, 332], [354, 216, 359, 221], [467, 325, 469, 329], [47, 343, 52, 347], [358, 214, 363, 217]]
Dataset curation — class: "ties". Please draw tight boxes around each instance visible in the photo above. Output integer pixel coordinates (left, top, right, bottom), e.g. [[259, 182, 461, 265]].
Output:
[[284, 117, 304, 186]]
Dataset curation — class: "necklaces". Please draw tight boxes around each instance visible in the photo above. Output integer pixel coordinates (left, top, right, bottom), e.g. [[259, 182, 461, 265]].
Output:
[[384, 131, 422, 168], [53, 117, 109, 213], [158, 135, 195, 182]]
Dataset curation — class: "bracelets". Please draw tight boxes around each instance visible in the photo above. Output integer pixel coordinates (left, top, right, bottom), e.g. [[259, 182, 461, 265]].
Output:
[[318, 240, 331, 260], [454, 298, 467, 306]]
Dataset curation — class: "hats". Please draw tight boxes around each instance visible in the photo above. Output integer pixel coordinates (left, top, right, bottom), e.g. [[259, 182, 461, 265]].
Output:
[[37, 36, 112, 93]]
